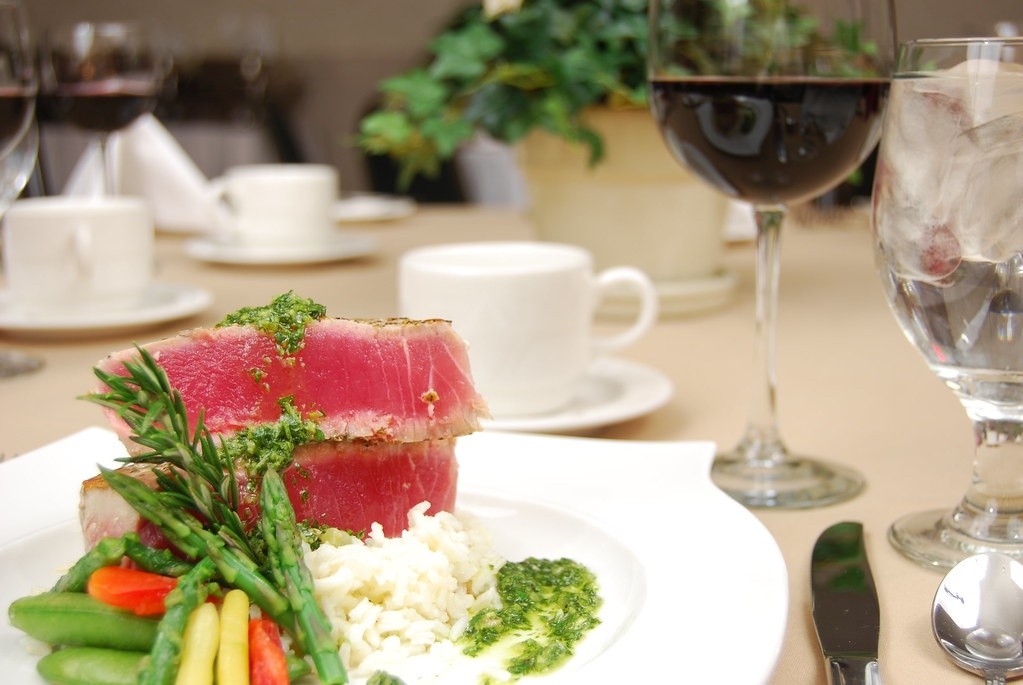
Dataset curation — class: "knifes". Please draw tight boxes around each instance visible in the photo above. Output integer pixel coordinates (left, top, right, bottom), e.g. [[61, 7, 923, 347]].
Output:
[[809, 520, 881, 685]]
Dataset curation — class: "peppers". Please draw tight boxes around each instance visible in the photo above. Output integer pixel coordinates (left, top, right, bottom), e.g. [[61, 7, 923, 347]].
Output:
[[87, 566, 223, 616], [247, 608, 291, 685]]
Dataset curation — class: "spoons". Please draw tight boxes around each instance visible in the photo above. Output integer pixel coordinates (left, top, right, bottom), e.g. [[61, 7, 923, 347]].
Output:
[[931, 551, 1023, 685]]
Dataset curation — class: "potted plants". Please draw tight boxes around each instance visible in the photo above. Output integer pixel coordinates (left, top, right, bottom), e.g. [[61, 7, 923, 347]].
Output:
[[352, 0, 940, 313]]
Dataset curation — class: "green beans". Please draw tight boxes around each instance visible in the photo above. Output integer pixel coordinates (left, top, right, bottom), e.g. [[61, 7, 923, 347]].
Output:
[[7, 590, 165, 684]]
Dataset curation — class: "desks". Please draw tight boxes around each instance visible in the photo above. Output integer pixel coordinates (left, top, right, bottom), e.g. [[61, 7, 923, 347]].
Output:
[[0, 204, 1023, 685]]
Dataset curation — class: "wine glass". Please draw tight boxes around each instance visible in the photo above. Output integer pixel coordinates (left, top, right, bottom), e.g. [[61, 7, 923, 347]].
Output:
[[648, 0, 898, 510], [39, 20, 163, 192], [871, 37, 1023, 573], [0, 0, 45, 384]]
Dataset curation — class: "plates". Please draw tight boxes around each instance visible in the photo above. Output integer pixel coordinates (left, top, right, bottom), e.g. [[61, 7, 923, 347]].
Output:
[[0, 426, 791, 685], [183, 238, 374, 265], [339, 190, 416, 222], [0, 281, 214, 338], [479, 359, 674, 437]]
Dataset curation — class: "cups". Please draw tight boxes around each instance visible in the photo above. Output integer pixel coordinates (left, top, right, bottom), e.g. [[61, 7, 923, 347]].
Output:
[[203, 162, 340, 246], [398, 242, 660, 414], [3, 194, 155, 309]]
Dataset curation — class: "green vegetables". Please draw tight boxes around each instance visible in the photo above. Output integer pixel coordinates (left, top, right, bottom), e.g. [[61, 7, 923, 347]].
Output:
[[459, 556, 602, 676], [212, 289, 370, 553], [77, 342, 268, 573]]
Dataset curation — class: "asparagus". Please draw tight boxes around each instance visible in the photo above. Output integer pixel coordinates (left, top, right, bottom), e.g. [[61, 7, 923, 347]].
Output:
[[29, 464, 348, 684]]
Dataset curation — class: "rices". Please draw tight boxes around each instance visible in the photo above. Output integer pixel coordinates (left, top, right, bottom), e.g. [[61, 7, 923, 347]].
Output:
[[252, 499, 504, 675]]
